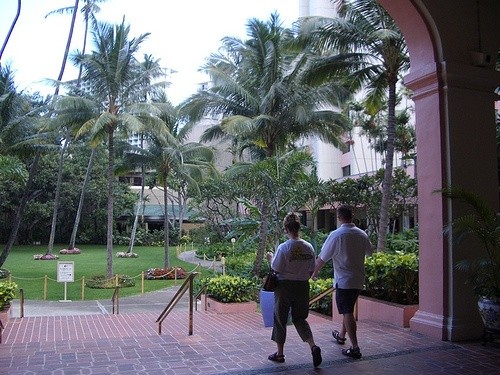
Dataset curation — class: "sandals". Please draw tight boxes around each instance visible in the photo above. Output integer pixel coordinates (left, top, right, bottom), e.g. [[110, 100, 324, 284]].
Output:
[[311, 345, 322, 367], [332, 330, 346, 345], [268, 352, 285, 363], [342, 346, 362, 358]]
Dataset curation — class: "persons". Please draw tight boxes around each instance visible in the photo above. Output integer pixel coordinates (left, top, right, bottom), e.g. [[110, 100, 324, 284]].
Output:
[[266, 213, 322, 367], [309, 204, 373, 358]]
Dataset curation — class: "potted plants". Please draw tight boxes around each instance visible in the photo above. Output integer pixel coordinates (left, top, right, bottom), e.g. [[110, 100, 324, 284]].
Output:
[[438, 171, 500, 332]]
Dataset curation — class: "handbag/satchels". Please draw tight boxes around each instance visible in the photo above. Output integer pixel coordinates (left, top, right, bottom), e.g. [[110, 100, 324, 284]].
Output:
[[263, 273, 276, 291]]
